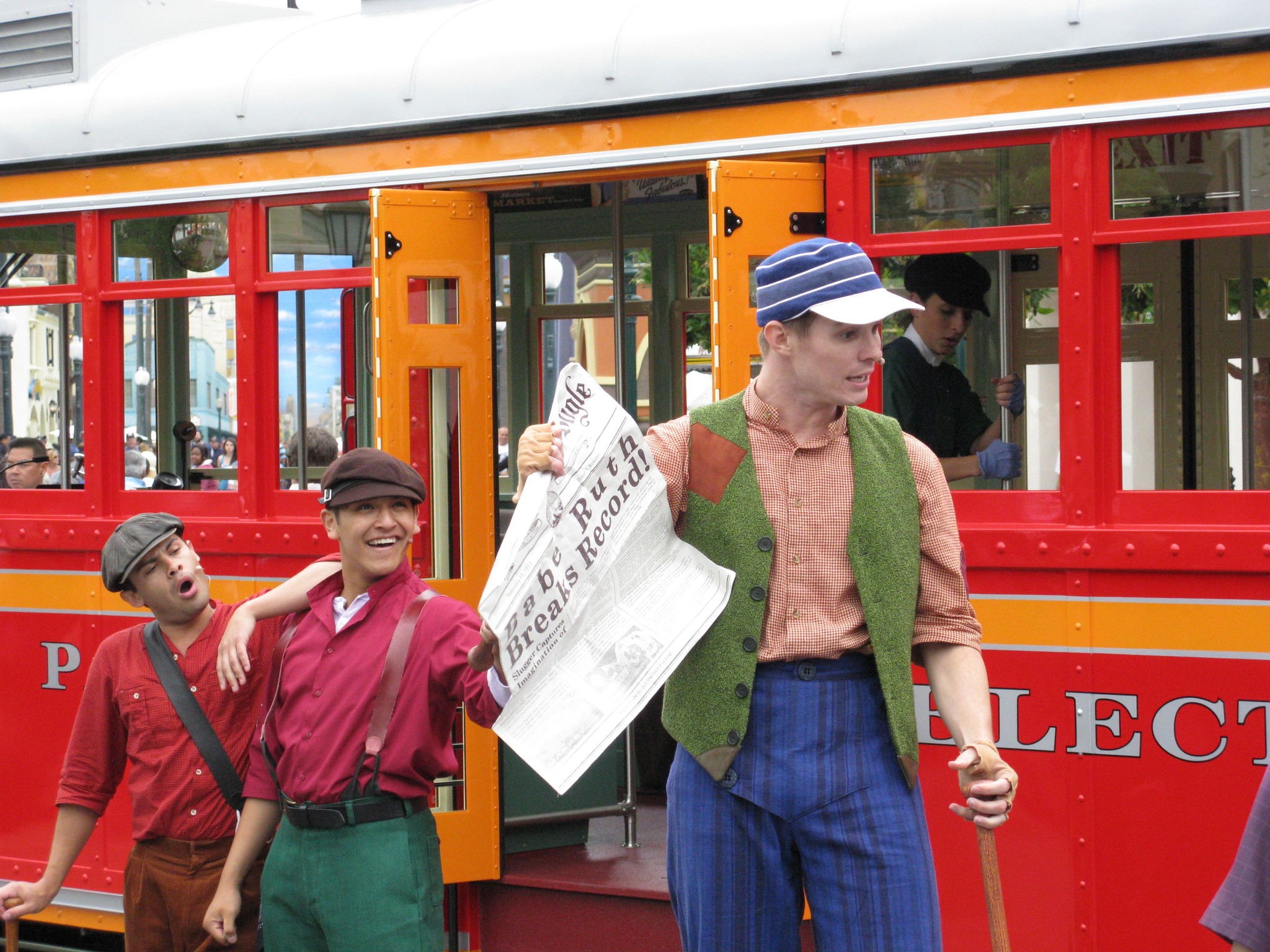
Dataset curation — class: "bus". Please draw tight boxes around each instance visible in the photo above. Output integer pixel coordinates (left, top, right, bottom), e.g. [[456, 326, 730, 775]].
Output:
[[0, 4, 1270, 950]]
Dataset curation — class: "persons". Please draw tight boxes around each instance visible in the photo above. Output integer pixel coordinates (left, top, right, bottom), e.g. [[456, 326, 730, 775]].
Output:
[[1197, 758, 1270, 946], [0, 510, 343, 952], [513, 236, 1020, 952], [1, 424, 338, 492], [200, 445, 516, 952], [882, 249, 1027, 485]]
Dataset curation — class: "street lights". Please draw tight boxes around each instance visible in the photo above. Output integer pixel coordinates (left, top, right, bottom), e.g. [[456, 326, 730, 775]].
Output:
[[133, 364, 151, 436], [68, 337, 84, 446]]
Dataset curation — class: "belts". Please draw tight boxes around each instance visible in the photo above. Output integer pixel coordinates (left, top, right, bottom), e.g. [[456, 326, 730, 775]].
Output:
[[282, 794, 429, 830]]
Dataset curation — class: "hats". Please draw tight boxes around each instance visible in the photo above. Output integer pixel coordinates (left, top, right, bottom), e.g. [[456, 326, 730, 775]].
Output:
[[904, 252, 994, 318], [102, 511, 185, 594], [755, 237, 926, 329], [319, 448, 427, 509]]
